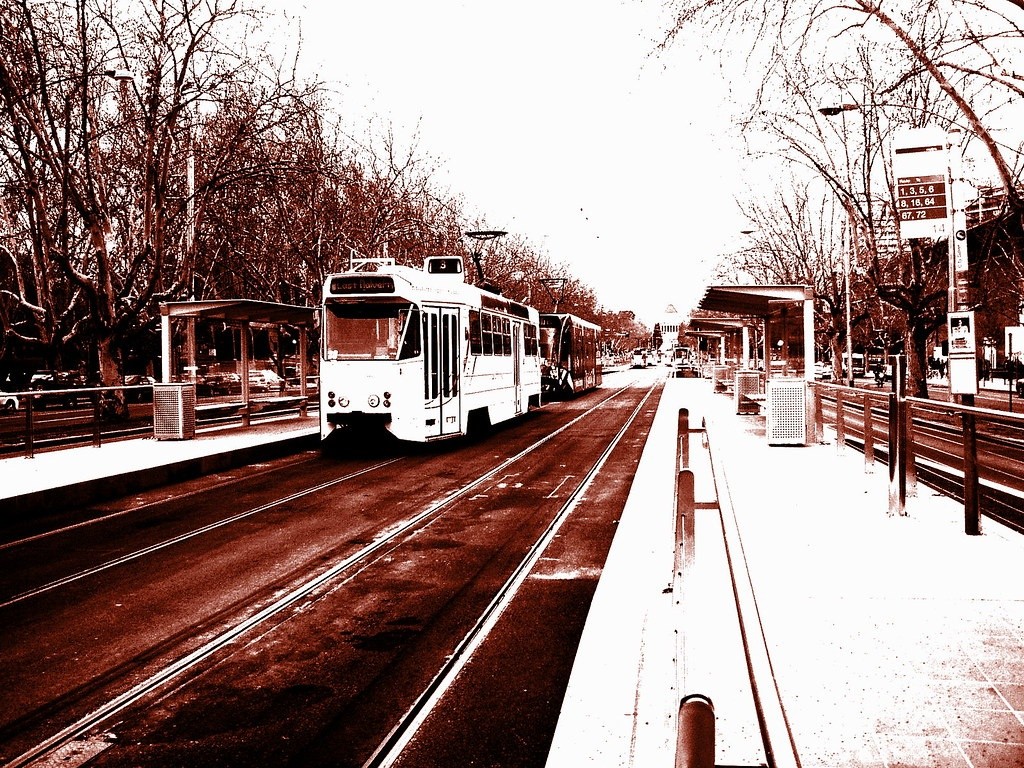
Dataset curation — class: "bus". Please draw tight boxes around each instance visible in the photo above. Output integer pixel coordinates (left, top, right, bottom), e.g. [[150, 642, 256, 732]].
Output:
[[310, 231, 543, 451], [526, 277, 602, 400]]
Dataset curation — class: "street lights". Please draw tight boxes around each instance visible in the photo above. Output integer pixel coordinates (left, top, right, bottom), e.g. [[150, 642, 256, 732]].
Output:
[[740, 208, 855, 388]]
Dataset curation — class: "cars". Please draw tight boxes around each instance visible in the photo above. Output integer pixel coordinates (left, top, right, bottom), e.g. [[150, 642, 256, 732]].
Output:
[[627, 345, 674, 369], [196, 371, 242, 397], [248, 369, 320, 394], [746, 350, 909, 384], [0, 368, 158, 421]]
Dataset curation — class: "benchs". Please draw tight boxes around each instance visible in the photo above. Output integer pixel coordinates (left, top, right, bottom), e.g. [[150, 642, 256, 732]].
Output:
[[196, 396, 309, 432], [744, 390, 766, 416], [717, 377, 734, 394]]
